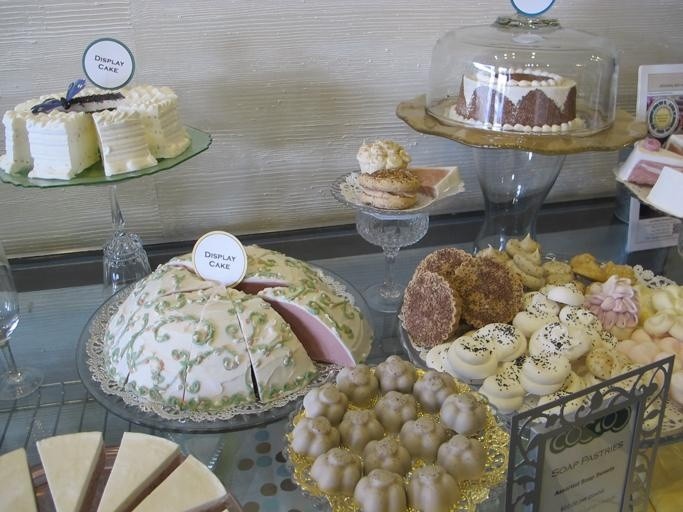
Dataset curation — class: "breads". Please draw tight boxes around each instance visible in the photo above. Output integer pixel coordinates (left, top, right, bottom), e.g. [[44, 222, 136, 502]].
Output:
[[1, 430, 227, 511]]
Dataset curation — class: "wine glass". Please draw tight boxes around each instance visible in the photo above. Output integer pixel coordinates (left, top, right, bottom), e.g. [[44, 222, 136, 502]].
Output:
[[353, 212, 430, 313], [0, 242, 45, 402]]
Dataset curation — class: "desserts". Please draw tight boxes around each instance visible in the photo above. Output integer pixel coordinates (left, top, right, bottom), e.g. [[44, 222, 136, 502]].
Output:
[[401, 234, 682, 431], [293, 354, 487, 511]]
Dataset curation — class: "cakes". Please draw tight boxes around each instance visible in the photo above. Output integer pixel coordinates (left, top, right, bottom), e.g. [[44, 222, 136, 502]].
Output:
[[356, 138, 458, 210], [0, 85, 192, 182], [103, 246, 369, 411], [618, 132, 683, 220], [445, 67, 586, 133]]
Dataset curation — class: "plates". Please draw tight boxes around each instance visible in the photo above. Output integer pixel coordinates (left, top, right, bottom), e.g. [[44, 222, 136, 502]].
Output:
[[329, 171, 434, 219], [281, 363, 509, 512], [0, 442, 242, 512], [73, 262, 374, 435], [397, 265, 682, 446]]
